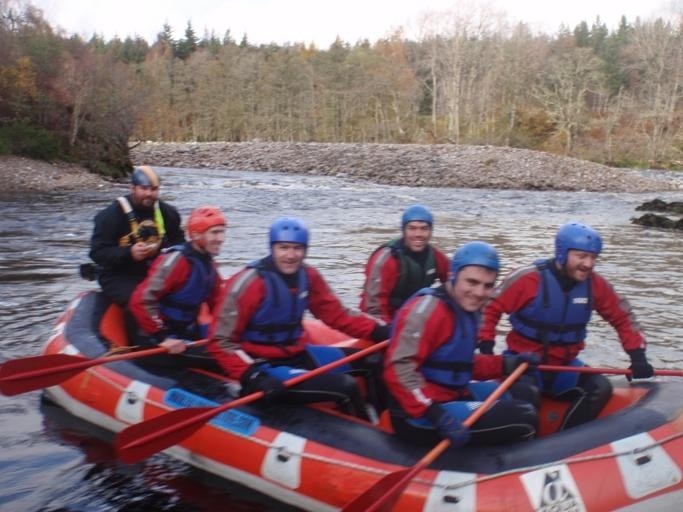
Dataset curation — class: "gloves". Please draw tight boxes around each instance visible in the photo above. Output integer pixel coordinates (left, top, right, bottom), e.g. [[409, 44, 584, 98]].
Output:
[[505, 354, 540, 381], [242, 364, 289, 408], [478, 340, 496, 355], [424, 405, 471, 450], [626, 349, 655, 381], [371, 325, 393, 344]]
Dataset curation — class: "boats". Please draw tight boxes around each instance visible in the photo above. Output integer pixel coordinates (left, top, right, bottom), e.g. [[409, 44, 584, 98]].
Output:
[[41, 290, 683, 512]]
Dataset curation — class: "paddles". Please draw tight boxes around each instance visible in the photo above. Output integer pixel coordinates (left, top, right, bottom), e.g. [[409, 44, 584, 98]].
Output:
[[0, 336, 212, 396], [116, 340, 394, 466], [340, 362, 529, 510]]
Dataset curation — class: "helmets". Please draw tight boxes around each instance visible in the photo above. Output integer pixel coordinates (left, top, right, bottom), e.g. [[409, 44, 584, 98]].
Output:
[[451, 242, 500, 287], [556, 222, 601, 266], [131, 164, 161, 190], [188, 206, 227, 248], [402, 207, 434, 232], [268, 218, 309, 248]]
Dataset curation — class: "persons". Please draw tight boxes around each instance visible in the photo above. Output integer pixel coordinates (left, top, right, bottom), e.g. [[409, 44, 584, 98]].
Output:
[[129, 205, 229, 395], [477, 223, 653, 439], [88, 165, 187, 345], [209, 218, 392, 422], [360, 204, 454, 326], [377, 242, 537, 451]]
